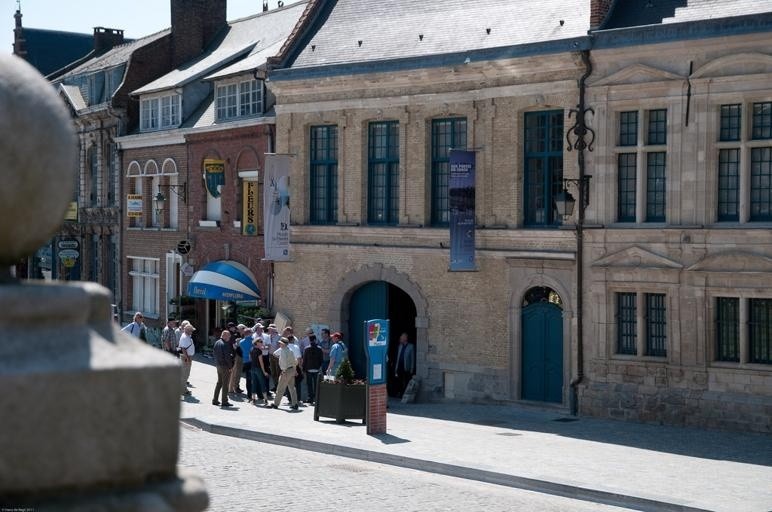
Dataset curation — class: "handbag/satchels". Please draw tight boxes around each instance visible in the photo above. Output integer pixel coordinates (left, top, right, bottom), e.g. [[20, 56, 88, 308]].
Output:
[[176, 346, 187, 357]]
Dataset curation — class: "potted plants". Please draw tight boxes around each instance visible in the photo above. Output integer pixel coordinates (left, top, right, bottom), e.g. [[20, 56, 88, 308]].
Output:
[[314, 358, 366, 425]]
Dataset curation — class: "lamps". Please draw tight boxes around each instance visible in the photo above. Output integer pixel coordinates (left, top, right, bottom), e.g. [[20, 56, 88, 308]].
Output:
[[555, 173, 592, 221], [152, 180, 186, 216]]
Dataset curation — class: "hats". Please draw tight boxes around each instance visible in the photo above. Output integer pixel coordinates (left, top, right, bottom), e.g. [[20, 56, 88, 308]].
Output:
[[303, 326, 314, 334], [277, 336, 290, 346], [252, 322, 265, 332], [330, 331, 343, 340], [184, 323, 197, 331], [166, 315, 180, 323], [266, 323, 279, 333]]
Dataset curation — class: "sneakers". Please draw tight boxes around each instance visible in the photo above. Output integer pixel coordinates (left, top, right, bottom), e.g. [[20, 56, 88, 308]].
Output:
[[211, 388, 316, 410], [181, 390, 193, 396]]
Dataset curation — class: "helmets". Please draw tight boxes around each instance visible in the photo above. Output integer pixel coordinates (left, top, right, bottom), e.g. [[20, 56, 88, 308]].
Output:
[[227, 321, 236, 328]]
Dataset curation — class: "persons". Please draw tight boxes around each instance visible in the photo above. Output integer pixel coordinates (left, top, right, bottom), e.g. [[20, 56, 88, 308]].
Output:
[[179, 325, 196, 396], [161, 317, 179, 354], [395, 333, 415, 396], [120, 312, 147, 340], [212, 323, 347, 410], [175, 320, 190, 344]]
